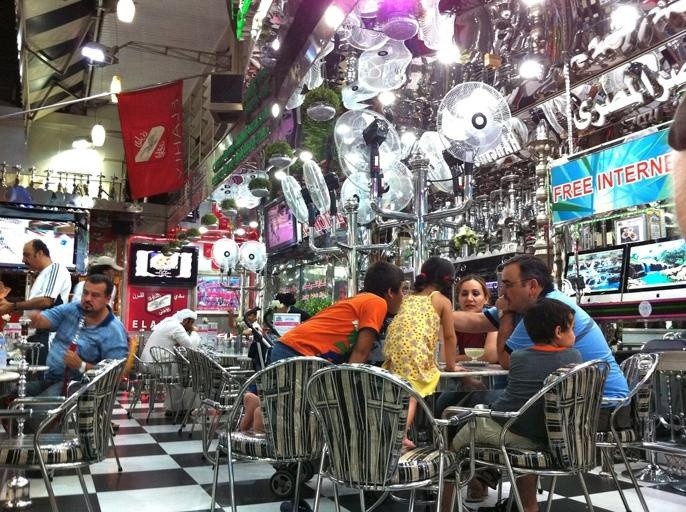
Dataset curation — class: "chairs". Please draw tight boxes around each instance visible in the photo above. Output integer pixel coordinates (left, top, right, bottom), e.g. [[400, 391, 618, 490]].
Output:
[[210, 355, 336, 512], [441, 358, 611, 511], [0, 332, 127, 512], [547, 350, 659, 512], [130, 333, 248, 467], [305, 363, 461, 511]]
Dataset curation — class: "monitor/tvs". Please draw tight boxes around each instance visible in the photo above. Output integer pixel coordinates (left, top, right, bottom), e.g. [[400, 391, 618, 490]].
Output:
[[564, 244, 626, 304], [0, 205, 91, 277], [128, 242, 198, 289], [263, 192, 302, 257], [623, 234, 686, 303]]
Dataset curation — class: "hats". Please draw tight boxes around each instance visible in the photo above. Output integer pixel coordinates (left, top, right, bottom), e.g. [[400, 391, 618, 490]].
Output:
[[91, 256, 124, 271]]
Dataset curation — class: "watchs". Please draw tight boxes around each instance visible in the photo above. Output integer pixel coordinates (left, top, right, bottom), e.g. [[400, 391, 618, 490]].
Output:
[[497, 310, 515, 320], [79, 360, 87, 373]]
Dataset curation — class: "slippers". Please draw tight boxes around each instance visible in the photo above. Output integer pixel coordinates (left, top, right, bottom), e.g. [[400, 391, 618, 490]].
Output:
[[280, 498, 313, 512]]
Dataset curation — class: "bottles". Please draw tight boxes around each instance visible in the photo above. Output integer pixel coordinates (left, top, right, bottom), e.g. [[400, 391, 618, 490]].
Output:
[[0, 338, 7, 371]]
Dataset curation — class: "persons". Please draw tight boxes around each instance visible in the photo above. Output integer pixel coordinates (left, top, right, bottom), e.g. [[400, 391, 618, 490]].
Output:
[[667, 92, 686, 241], [233, 309, 263, 338], [444, 255, 632, 511], [380, 256, 468, 448], [10, 274, 128, 433], [0, 239, 128, 333], [139, 308, 201, 417], [274, 292, 311, 326], [239, 261, 405, 432], [435, 275, 503, 512], [442, 297, 581, 512]]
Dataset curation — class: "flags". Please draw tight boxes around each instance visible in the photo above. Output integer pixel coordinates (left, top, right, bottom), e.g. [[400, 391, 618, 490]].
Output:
[[118, 79, 185, 201]]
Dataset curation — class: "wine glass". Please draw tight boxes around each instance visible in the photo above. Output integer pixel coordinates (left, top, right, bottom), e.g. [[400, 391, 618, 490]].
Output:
[[460, 346, 490, 366]]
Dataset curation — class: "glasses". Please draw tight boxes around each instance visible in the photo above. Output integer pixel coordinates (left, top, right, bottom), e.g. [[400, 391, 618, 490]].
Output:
[[501, 277, 540, 288]]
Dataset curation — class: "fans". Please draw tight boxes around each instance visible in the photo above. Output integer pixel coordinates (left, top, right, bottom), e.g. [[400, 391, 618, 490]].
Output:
[[211, 0, 512, 321]]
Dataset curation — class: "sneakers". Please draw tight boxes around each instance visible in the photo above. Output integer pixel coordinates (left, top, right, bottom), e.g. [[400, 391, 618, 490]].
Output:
[[478, 498, 519, 512]]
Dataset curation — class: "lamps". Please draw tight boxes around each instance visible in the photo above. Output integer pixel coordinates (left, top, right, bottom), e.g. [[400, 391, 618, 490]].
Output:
[[81, 42, 232, 70]]
